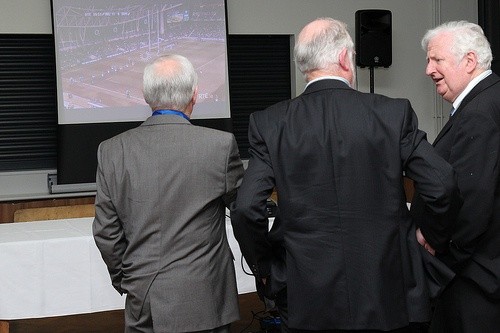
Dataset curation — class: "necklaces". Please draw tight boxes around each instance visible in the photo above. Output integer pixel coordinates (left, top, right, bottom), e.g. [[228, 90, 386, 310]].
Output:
[[410, 20, 500, 333]]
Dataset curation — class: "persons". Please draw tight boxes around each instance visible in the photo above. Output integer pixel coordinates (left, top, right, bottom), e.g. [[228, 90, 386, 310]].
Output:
[[92, 55, 245, 333], [57, 6, 229, 106], [230, 19, 452, 333]]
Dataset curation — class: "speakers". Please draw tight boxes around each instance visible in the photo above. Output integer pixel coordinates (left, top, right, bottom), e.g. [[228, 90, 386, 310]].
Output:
[[355, 10, 393, 69]]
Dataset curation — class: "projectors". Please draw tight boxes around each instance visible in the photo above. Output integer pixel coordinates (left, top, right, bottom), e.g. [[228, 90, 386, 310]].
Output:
[[226, 197, 276, 218]]
